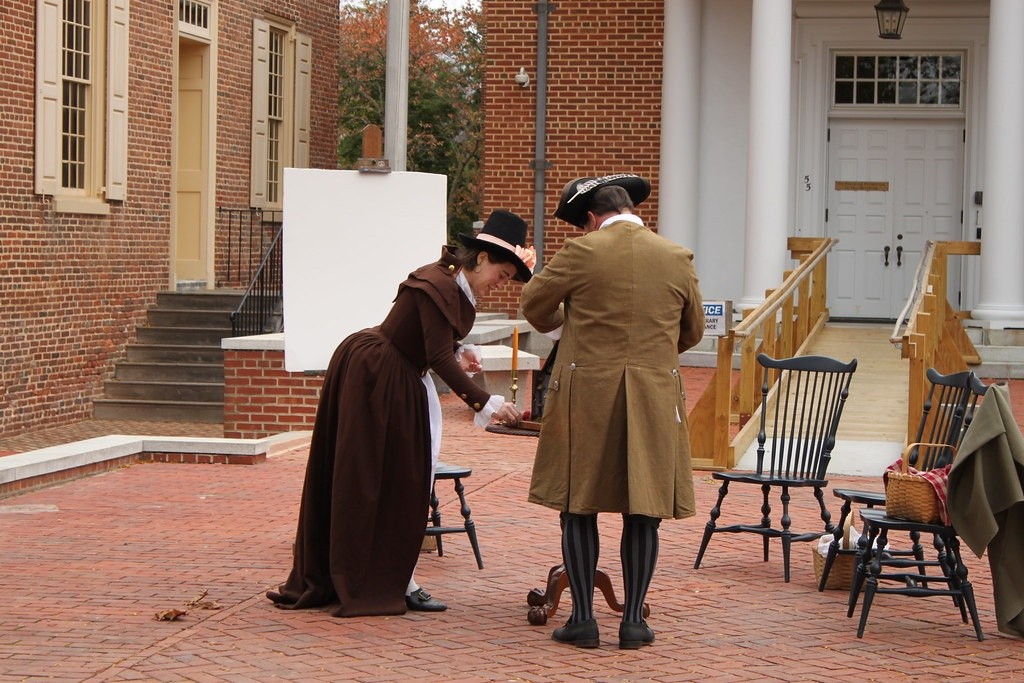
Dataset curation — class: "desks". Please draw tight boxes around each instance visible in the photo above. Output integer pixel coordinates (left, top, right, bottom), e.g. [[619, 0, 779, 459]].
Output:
[[485, 418, 650, 626]]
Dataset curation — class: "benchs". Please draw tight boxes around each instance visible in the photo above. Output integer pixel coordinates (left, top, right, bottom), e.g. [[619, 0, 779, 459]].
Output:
[[429, 307, 554, 420]]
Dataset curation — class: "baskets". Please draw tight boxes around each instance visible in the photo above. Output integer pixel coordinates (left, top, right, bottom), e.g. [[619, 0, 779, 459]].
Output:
[[812, 510, 867, 591], [886, 443, 959, 523]]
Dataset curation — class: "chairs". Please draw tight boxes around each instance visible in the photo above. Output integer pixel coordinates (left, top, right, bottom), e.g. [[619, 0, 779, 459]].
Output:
[[692, 351, 858, 583], [820, 370, 1011, 642], [423, 466, 485, 570]]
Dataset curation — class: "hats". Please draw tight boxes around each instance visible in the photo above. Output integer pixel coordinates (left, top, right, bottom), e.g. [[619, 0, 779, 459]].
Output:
[[553, 173, 651, 228], [459, 208, 537, 282]]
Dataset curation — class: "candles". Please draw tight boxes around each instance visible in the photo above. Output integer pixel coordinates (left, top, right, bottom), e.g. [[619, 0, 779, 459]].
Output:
[[512, 326, 518, 371]]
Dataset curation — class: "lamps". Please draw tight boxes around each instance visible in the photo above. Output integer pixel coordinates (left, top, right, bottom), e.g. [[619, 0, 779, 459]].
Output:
[[873, 0, 910, 39]]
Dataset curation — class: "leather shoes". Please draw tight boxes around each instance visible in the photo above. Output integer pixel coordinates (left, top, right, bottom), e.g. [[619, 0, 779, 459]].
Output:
[[619, 618, 655, 648], [405, 586, 447, 611], [551, 614, 600, 647]]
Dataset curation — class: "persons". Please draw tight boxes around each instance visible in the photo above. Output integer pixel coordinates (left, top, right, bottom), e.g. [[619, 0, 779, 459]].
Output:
[[268, 209, 536, 617], [521, 173, 706, 649]]
